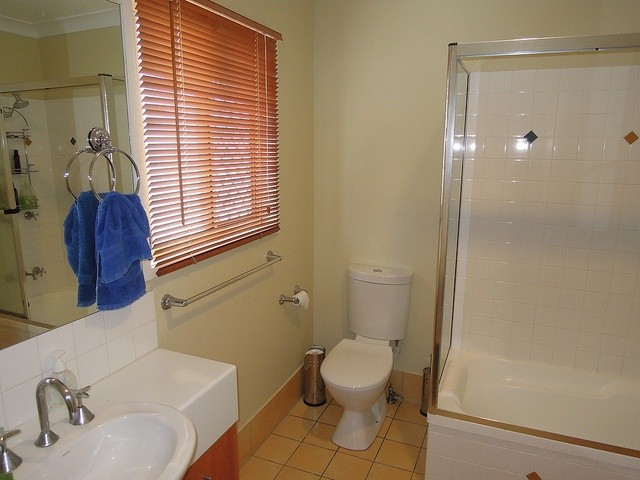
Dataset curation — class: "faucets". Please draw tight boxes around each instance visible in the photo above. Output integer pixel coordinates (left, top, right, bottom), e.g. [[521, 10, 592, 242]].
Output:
[[34, 378, 76, 448], [25, 272, 39, 283]]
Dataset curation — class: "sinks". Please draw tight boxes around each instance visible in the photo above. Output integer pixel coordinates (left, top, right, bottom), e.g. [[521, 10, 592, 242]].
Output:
[[30, 403, 196, 479]]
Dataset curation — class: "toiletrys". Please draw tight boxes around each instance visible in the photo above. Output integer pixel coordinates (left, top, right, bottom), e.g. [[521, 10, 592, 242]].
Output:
[[17, 175, 38, 212], [13, 147, 22, 173], [51, 347, 77, 407]]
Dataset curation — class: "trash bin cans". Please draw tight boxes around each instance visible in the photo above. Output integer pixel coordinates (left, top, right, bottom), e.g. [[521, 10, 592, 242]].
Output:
[[303, 345, 326, 406]]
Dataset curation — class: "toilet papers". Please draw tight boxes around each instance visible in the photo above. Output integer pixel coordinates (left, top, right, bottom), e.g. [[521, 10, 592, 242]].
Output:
[[292, 290, 309, 312]]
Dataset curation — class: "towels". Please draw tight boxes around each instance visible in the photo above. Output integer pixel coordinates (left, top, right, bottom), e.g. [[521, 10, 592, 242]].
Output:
[[64, 190, 96, 308], [95, 192, 153, 311]]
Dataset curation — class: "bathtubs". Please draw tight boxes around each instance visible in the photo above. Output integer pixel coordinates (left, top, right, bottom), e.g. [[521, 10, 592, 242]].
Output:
[[27, 288, 96, 326], [425, 351, 640, 480]]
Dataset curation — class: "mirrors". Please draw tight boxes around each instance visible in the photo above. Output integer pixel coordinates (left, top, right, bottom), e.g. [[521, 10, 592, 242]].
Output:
[[0, 0, 135, 349]]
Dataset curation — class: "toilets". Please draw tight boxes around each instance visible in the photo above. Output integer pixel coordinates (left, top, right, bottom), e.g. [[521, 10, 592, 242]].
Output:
[[319, 263, 416, 451]]
